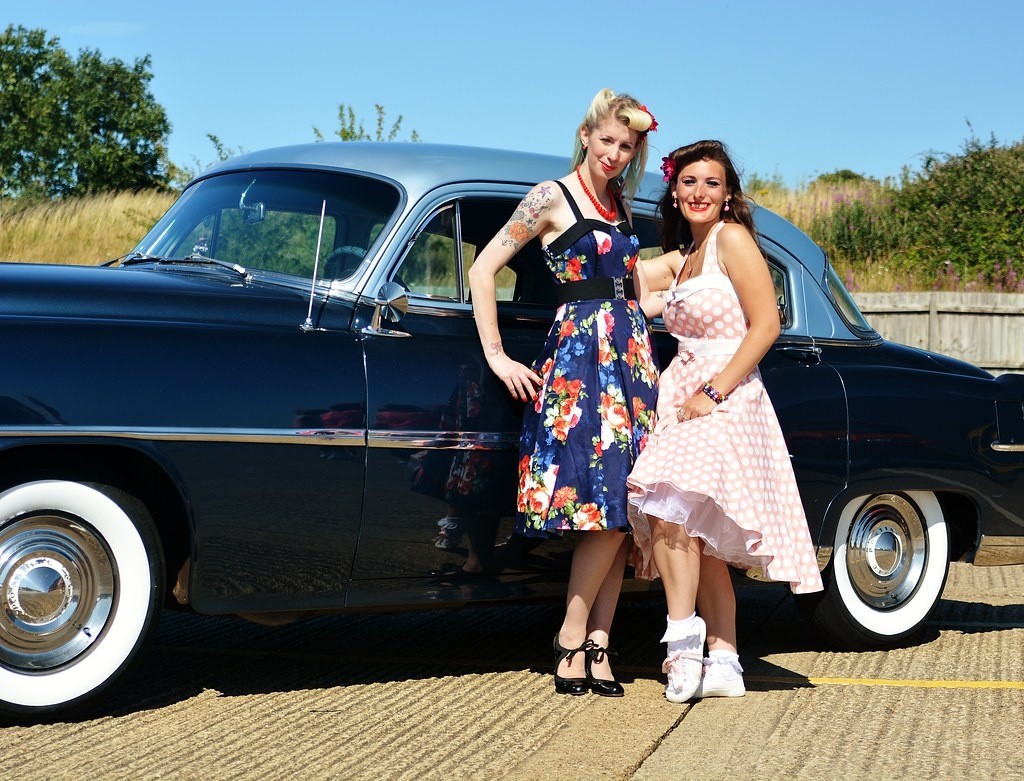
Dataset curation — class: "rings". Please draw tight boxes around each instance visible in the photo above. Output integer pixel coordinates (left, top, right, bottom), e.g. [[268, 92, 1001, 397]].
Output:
[[679, 408, 684, 414], [682, 416, 688, 421]]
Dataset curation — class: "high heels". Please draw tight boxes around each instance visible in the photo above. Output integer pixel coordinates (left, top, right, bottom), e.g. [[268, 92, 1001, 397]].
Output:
[[553, 632, 624, 697]]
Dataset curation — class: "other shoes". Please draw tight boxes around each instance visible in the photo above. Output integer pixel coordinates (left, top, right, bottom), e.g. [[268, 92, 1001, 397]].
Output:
[[660, 616, 706, 702], [693, 648, 745, 697]]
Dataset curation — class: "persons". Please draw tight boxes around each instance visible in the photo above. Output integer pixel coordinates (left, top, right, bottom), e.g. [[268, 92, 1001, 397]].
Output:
[[468, 87, 670, 698], [624, 140, 823, 704]]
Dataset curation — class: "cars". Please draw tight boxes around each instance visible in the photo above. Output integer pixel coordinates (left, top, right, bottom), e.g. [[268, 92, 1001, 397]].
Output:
[[0, 140, 1024, 729]]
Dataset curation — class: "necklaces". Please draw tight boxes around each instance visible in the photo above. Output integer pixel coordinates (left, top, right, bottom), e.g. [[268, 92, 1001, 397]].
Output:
[[687, 223, 719, 279]]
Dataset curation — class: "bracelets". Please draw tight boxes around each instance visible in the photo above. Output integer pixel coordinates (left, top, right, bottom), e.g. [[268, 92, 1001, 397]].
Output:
[[701, 382, 729, 404]]
